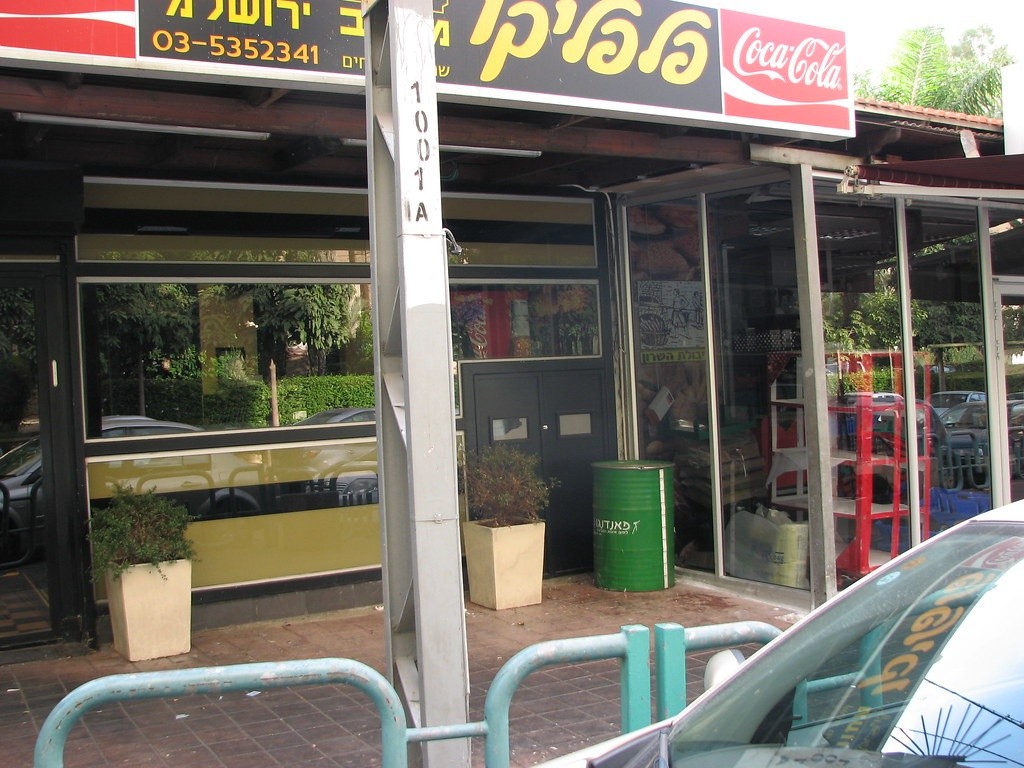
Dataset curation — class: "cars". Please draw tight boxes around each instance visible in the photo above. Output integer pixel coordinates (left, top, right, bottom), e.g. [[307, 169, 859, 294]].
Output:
[[520, 495, 1024, 768], [269, 405, 377, 482], [0, 414, 263, 568], [838, 389, 1024, 505]]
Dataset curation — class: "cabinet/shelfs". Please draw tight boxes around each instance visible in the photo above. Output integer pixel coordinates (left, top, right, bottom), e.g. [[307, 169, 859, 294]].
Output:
[[768, 345, 936, 584]]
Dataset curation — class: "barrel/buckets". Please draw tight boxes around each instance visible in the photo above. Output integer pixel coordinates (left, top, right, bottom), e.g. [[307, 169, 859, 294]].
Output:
[[590, 461, 676, 592]]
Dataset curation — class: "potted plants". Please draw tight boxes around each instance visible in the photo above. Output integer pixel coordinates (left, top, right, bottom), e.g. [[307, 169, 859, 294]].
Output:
[[87, 481, 196, 660], [460, 442, 562, 610]]
